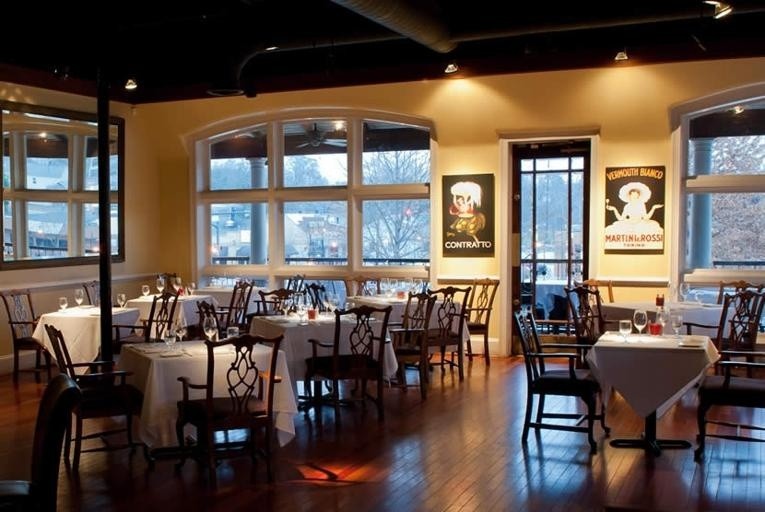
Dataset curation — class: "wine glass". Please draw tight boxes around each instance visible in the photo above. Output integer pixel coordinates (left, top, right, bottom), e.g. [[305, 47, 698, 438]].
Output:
[[632, 309, 649, 344], [670, 312, 685, 338], [57, 276, 196, 313], [619, 320, 632, 344], [280, 291, 355, 327], [163, 316, 187, 353], [658, 308, 672, 337], [203, 316, 217, 342], [379, 277, 423, 301]]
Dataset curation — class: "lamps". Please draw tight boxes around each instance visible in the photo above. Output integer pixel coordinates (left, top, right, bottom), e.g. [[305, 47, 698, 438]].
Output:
[[124, 79, 137, 91], [443, 63, 459, 75], [704, 1, 734, 20], [615, 50, 628, 62]]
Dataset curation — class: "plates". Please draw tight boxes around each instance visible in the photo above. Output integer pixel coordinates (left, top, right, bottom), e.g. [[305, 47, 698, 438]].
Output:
[[677, 342, 705, 347]]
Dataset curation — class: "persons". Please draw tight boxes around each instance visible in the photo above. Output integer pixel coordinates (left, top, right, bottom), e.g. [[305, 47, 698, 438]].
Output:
[[606, 182, 664, 249], [449, 190, 486, 243]]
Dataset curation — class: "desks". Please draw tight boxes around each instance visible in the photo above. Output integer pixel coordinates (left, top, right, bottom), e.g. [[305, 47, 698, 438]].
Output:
[[586, 331, 720, 460]]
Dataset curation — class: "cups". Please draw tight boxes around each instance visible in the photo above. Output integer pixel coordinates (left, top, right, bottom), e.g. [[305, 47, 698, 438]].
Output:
[[656, 292, 665, 309], [649, 318, 663, 337], [226, 327, 240, 349]]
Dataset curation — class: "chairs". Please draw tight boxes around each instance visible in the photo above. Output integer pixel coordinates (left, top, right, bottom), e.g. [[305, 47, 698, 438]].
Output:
[[691, 350, 765, 457], [2, 375, 84, 511], [301, 305, 393, 429], [517, 304, 611, 457], [684, 292, 765, 376], [560, 285, 612, 369], [172, 331, 285, 490], [519, 277, 765, 337], [44, 324, 156, 477], [2, 272, 500, 382], [355, 290, 438, 401]]
